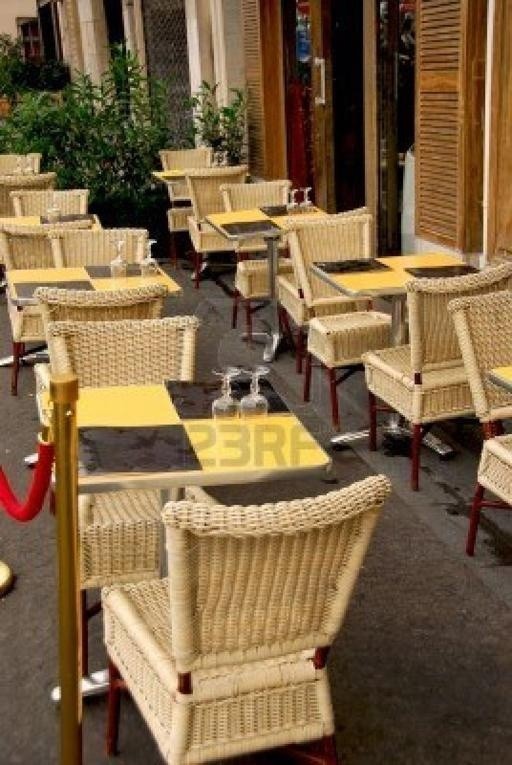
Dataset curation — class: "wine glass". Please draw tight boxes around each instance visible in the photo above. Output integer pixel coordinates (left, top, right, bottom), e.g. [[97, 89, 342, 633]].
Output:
[[109, 238, 157, 281], [287, 187, 313, 213], [211, 364, 271, 419]]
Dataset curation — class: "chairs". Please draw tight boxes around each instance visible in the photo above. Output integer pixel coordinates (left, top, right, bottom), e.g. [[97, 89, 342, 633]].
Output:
[[1, 147, 512, 764]]
